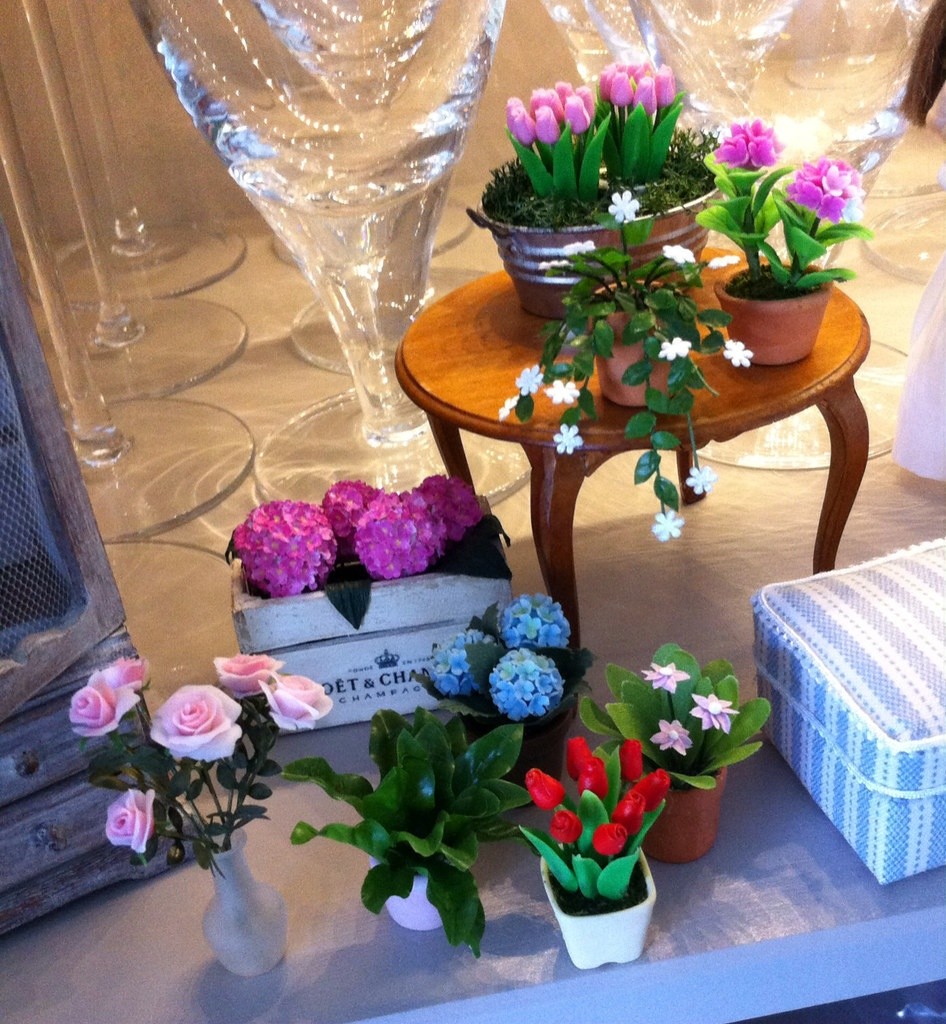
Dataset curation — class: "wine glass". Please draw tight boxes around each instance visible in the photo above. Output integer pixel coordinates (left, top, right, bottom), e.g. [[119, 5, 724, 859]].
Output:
[[0, 0, 946, 543]]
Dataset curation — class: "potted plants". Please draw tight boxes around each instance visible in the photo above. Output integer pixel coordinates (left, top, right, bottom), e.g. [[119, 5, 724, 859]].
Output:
[[279, 704, 532, 957]]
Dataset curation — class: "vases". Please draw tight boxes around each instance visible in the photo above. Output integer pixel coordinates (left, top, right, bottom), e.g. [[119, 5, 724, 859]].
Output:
[[711, 263, 832, 364], [622, 765, 729, 866], [583, 280, 699, 407], [198, 826, 290, 979], [541, 844, 656, 970], [461, 184, 723, 325]]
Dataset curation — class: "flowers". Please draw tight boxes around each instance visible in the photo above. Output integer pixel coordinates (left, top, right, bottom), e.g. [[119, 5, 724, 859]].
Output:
[[416, 587, 586, 719], [496, 46, 875, 540], [516, 723, 671, 897], [572, 630, 769, 791], [67, 651, 335, 877], [213, 461, 486, 622]]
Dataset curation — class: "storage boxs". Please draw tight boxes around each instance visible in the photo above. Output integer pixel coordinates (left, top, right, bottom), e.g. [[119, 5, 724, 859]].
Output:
[[229, 559, 514, 736]]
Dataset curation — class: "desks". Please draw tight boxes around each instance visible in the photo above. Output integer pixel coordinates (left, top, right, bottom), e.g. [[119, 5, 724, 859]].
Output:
[[383, 246, 875, 683]]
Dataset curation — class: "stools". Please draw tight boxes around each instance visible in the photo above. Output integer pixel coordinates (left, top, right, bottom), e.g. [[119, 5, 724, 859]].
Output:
[[750, 534, 946, 887]]
